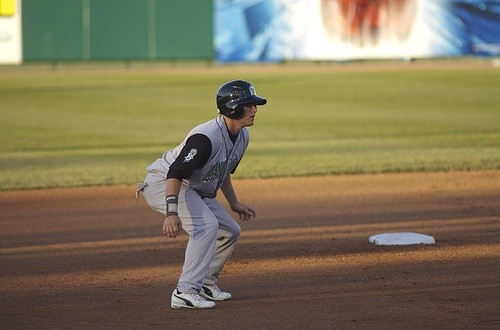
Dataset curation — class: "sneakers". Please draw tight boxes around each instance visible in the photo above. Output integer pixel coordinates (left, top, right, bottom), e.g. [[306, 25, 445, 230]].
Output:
[[171, 289, 216, 309], [199, 285, 232, 300]]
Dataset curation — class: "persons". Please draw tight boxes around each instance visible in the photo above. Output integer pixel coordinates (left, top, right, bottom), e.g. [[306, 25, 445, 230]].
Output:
[[141, 79, 267, 309]]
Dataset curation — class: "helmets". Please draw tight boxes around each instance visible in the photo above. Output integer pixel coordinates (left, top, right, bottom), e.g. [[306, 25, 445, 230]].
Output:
[[216, 80, 267, 119]]
